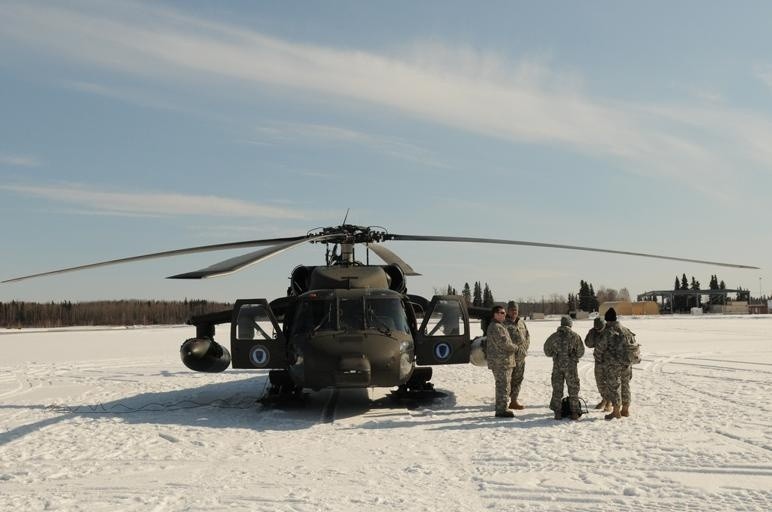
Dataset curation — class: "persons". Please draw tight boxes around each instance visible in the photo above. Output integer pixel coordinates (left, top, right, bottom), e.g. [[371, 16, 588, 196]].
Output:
[[594, 307, 637, 420], [485, 305, 519, 418], [585, 316, 615, 412], [504, 301, 530, 410], [543, 316, 584, 421]]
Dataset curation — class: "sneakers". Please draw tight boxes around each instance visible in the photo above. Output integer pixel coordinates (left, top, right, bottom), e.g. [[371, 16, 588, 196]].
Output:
[[508, 401, 524, 409], [495, 411, 515, 418]]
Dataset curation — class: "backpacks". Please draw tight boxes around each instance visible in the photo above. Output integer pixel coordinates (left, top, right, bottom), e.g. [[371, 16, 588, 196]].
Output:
[[551, 396, 589, 421], [615, 326, 642, 365], [543, 330, 565, 357]]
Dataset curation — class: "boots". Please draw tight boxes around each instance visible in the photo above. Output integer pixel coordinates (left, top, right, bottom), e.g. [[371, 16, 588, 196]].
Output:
[[620, 404, 631, 418], [603, 405, 622, 420], [594, 399, 613, 412]]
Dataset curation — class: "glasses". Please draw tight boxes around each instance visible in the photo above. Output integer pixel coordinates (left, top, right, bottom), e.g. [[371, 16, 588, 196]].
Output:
[[495, 311, 506, 315]]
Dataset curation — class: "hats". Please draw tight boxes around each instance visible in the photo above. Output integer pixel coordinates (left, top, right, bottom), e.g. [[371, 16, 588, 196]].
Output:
[[593, 317, 606, 330], [507, 301, 520, 308], [560, 315, 573, 327], [605, 308, 619, 322]]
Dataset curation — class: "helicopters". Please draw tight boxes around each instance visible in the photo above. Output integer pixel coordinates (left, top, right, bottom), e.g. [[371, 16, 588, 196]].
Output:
[[1, 206, 761, 405]]
